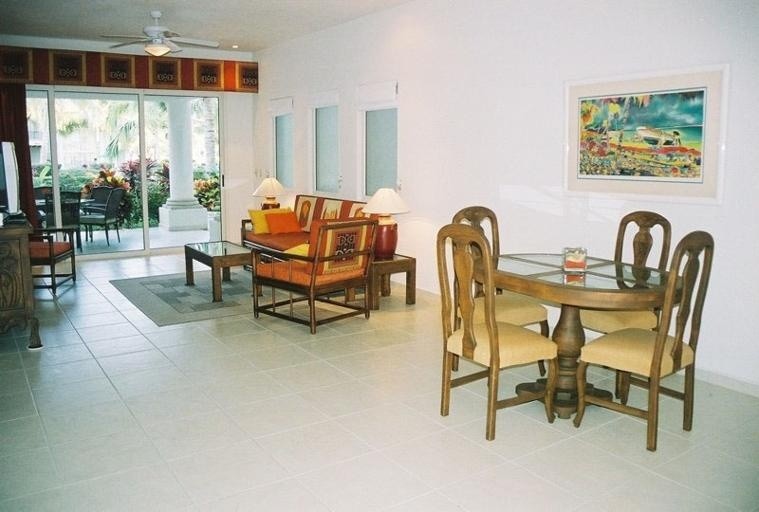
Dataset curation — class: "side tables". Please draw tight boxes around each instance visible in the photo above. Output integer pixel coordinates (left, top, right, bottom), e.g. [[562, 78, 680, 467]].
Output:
[[345, 254, 416, 310]]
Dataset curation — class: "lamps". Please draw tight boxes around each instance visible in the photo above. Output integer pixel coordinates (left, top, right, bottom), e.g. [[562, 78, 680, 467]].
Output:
[[360, 188, 411, 261], [252, 177, 284, 210], [144, 39, 171, 57]]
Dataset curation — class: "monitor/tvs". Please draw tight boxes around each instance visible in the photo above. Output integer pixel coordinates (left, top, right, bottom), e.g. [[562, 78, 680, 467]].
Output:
[[0, 141, 22, 215]]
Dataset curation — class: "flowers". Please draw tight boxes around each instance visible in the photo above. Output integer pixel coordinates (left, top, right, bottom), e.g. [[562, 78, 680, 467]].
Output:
[[193, 179, 220, 211]]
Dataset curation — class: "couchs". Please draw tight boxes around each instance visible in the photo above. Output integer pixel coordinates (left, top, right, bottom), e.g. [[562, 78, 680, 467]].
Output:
[[241, 195, 380, 273], [251, 219, 379, 334]]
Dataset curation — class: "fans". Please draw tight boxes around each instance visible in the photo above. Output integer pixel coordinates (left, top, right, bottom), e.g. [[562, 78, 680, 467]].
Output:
[[100, 10, 220, 53]]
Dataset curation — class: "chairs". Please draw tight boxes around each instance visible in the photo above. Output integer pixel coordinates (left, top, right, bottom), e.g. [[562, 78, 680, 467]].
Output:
[[33, 186, 124, 252], [29, 228, 76, 294], [451, 206, 549, 376], [437, 224, 560, 441], [579, 211, 672, 398], [573, 231, 714, 452]]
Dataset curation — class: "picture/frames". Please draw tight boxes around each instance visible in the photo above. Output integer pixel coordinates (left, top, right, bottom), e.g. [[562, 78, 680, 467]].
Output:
[[563, 62, 730, 206]]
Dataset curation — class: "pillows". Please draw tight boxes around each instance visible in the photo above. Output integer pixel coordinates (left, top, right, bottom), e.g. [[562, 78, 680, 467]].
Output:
[[265, 212, 303, 235], [283, 243, 309, 264], [248, 207, 293, 235]]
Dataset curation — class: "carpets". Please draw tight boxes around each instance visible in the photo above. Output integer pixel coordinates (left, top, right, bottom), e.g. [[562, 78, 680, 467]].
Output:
[[109, 270, 292, 327]]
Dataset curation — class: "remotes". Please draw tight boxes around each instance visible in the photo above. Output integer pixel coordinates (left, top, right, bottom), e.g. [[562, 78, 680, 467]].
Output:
[[8, 219, 27, 224]]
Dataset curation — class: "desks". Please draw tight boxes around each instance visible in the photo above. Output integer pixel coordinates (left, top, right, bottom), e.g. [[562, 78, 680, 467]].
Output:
[[0, 220, 43, 348]]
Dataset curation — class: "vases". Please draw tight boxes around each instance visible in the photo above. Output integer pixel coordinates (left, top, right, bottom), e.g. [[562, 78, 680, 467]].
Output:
[[207, 212, 222, 241]]
